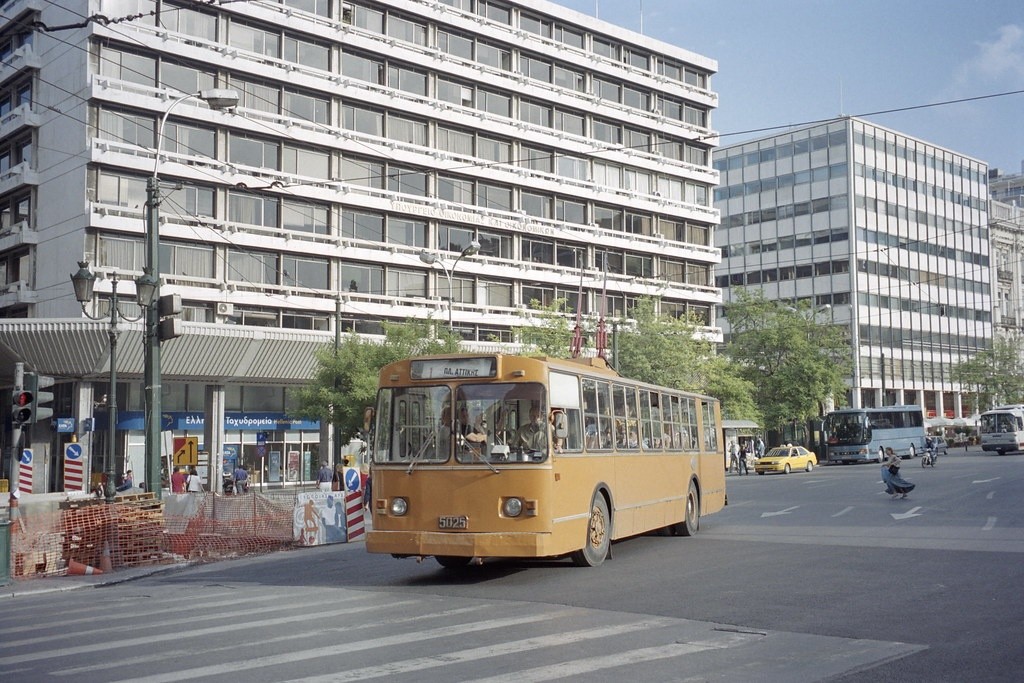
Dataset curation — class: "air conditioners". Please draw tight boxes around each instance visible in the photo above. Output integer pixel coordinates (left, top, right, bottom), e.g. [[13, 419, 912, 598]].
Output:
[[192, 155, 209, 168], [513, 210, 526, 220], [216, 303, 234, 315], [101, 266, 120, 279], [513, 303, 526, 315], [652, 109, 663, 161], [194, 213, 215, 230], [587, 179, 596, 186], [163, 88, 180, 99], [431, 46, 442, 58], [414, 296, 442, 308], [590, 312, 599, 321], [513, 71, 524, 81], [588, 267, 598, 275], [331, 178, 344, 188], [654, 234, 664, 241], [258, 167, 276, 178], [653, 192, 663, 199], [589, 223, 599, 231]]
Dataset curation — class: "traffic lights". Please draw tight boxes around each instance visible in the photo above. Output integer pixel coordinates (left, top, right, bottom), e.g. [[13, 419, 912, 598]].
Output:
[[24, 375, 54, 422], [12, 390, 32, 424]]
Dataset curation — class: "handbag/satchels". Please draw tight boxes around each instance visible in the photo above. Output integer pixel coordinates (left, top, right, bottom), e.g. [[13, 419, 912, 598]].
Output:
[[186, 483, 189, 492], [232, 485, 237, 496], [889, 464, 900, 476]]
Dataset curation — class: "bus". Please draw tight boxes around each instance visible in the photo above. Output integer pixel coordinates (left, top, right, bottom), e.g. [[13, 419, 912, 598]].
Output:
[[821, 405, 928, 464], [979, 404, 1024, 455], [365, 352, 729, 564]]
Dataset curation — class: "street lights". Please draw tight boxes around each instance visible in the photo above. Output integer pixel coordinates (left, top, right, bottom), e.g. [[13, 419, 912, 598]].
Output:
[[69, 258, 162, 502], [784, 303, 832, 445], [141, 89, 242, 496], [418, 242, 481, 350]]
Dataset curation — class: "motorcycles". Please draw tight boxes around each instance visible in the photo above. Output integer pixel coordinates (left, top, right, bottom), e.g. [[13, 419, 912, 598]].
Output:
[[919, 446, 937, 468]]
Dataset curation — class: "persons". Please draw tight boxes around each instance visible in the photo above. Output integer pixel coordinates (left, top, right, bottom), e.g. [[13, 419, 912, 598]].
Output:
[[924, 437, 935, 460], [603, 426, 671, 449], [363, 467, 372, 513], [728, 440, 740, 474], [186, 469, 202, 492], [756, 440, 764, 458], [440, 406, 487, 451], [171, 467, 185, 494], [116, 470, 133, 491], [235, 465, 248, 495], [881, 447, 915, 499], [737, 444, 749, 476], [316, 461, 333, 492], [511, 406, 548, 450]]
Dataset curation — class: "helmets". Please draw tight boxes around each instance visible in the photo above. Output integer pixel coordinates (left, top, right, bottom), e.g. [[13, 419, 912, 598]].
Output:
[[926, 437, 932, 444]]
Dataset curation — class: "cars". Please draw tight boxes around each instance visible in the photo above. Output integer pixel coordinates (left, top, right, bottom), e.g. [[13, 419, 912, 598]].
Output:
[[927, 436, 949, 454], [754, 443, 817, 475]]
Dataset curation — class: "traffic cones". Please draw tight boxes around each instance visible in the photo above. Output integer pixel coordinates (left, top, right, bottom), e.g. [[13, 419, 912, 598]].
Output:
[[100, 541, 114, 574], [67, 559, 104, 576]]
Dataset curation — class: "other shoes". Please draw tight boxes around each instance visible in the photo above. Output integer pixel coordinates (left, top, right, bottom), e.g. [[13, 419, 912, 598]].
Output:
[[892, 494, 898, 498], [900, 494, 908, 499]]
